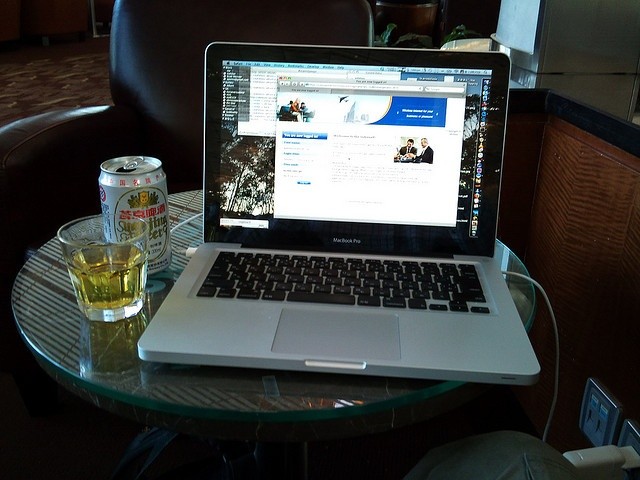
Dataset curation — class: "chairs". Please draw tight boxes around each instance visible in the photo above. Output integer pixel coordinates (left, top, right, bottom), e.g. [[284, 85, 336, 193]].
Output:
[[0, 0, 373, 221]]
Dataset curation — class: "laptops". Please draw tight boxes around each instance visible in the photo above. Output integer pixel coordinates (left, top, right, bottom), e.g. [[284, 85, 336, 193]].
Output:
[[136, 40, 542, 387]]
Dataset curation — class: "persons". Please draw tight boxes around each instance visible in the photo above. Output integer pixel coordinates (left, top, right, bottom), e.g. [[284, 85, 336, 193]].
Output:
[[289, 98, 302, 123], [288, 101, 293, 107], [397, 139, 418, 159], [408, 138, 434, 163], [300, 102, 306, 109]]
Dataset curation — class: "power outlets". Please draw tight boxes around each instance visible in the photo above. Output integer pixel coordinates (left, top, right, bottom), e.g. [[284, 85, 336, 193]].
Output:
[[577, 376, 617, 449], [615, 419, 640, 453]]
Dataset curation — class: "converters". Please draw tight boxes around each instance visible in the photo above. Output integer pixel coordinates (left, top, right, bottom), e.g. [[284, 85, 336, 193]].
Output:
[[562, 444, 640, 479]]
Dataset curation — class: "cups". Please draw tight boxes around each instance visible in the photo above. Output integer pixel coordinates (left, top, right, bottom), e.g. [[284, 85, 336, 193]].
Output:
[[57, 213, 149, 322], [78, 308, 160, 382]]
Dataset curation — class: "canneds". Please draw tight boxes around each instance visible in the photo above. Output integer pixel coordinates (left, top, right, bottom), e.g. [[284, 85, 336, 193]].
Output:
[[98, 156, 172, 277]]
